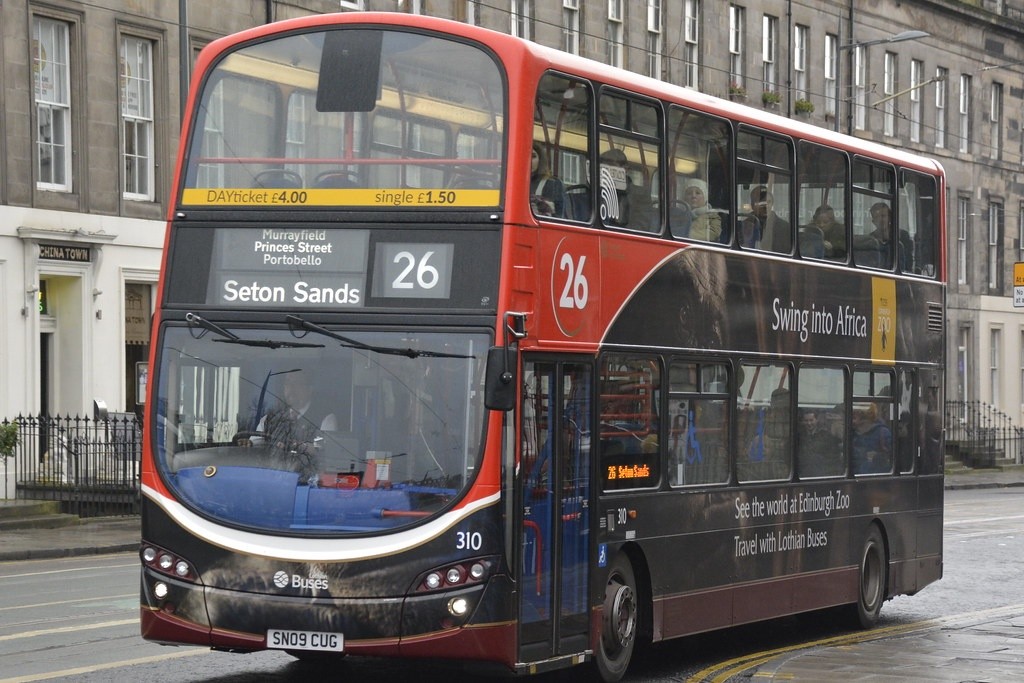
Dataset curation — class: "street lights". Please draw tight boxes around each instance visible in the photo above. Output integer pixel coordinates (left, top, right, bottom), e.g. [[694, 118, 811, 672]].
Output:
[[254, 367, 304, 430]]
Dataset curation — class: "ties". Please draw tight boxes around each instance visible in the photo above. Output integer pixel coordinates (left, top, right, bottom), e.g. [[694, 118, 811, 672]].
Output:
[[291, 409, 299, 422]]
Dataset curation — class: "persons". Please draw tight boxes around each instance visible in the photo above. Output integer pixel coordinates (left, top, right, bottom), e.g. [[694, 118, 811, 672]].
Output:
[[747, 388, 792, 480], [600, 149, 651, 232], [238, 368, 337, 452], [742, 185, 789, 252], [530, 143, 561, 217], [870, 203, 913, 273], [799, 404, 892, 473], [807, 204, 845, 259], [679, 179, 721, 239]]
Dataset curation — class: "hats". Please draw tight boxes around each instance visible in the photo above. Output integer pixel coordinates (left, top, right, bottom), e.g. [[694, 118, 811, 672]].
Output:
[[684, 178, 708, 204]]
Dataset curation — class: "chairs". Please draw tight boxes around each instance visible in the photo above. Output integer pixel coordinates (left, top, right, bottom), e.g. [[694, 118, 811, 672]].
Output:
[[448, 174, 909, 272]]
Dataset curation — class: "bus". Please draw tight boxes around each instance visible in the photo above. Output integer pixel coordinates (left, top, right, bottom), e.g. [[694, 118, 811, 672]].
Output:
[[140, 10, 947, 683]]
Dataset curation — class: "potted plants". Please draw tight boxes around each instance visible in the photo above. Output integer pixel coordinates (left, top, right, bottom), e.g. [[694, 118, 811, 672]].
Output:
[[729, 82, 749, 104], [761, 90, 784, 111], [794, 98, 816, 118], [825, 110, 835, 122]]
